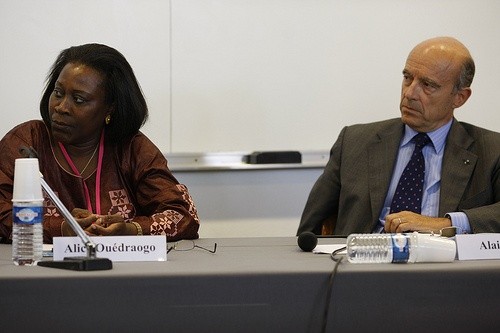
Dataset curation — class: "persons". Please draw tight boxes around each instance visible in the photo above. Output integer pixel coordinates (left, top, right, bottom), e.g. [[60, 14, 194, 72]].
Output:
[[296, 37, 500, 237], [0, 43, 200, 245]]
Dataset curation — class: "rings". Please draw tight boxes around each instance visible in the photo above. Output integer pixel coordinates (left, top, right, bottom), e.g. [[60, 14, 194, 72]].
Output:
[[398, 217, 401, 223]]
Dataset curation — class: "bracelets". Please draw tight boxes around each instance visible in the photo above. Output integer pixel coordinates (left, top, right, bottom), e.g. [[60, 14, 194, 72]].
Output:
[[132, 222, 143, 236]]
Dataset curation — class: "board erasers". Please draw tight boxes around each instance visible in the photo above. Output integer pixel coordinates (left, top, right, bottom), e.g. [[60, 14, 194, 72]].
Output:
[[241, 151, 301, 166]]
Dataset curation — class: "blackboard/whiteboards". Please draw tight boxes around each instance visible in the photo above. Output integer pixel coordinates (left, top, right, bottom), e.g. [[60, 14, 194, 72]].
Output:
[[1, 1, 498, 173]]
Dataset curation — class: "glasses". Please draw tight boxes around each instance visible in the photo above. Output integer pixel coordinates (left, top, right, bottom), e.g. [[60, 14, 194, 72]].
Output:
[[167, 240, 217, 254], [417, 227, 467, 237]]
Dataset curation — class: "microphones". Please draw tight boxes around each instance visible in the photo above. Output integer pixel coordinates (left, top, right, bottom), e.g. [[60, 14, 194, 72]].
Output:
[[16, 142, 112, 271], [298, 232, 348, 252]]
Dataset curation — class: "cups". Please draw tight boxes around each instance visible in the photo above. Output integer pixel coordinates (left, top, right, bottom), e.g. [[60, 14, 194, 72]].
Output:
[[412, 230, 456, 263], [12, 157, 45, 202]]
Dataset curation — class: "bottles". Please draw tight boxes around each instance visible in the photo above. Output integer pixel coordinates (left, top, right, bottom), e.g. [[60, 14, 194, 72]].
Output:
[[12, 201, 45, 266], [346, 232, 417, 264]]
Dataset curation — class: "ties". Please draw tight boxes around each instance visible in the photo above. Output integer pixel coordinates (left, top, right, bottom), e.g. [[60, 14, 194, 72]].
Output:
[[389, 133, 431, 232]]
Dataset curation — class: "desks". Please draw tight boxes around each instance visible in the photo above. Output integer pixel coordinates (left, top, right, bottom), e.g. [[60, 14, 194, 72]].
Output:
[[0, 237, 499, 333]]
